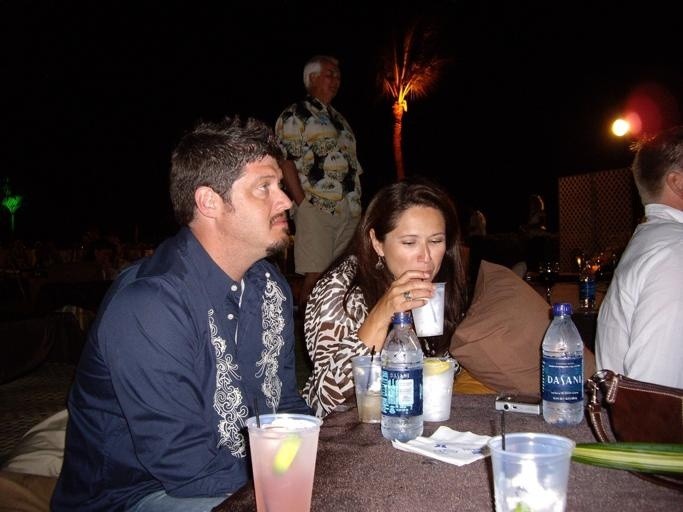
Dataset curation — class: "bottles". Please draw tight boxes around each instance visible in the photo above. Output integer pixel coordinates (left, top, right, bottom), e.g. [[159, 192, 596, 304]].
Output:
[[381, 310, 423, 443], [537, 304, 586, 423], [580, 260, 597, 309]]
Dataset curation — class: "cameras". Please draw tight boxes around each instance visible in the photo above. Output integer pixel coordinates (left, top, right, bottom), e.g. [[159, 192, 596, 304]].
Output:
[[494, 394, 542, 416]]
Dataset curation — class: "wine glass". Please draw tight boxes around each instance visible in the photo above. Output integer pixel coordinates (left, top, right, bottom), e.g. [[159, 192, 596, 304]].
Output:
[[537, 262, 560, 305]]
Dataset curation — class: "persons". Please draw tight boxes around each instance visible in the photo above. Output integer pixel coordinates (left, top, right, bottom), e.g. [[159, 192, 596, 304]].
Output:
[[593, 122, 683, 392], [272, 53, 364, 321], [298, 173, 467, 422], [519, 193, 547, 233], [47, 112, 316, 511], [466, 198, 486, 237]]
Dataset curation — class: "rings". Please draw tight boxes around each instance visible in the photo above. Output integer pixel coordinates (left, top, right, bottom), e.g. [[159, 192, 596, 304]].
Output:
[[403, 290, 412, 300]]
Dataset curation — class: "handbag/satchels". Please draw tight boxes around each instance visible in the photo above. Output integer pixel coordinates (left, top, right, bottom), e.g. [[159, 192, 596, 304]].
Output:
[[584, 369, 683, 444]]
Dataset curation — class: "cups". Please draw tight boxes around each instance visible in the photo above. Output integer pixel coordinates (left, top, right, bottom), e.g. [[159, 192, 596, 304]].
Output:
[[486, 431, 573, 512], [351, 356, 384, 422], [244, 413, 323, 511], [421, 356, 456, 422], [406, 280, 448, 338]]
